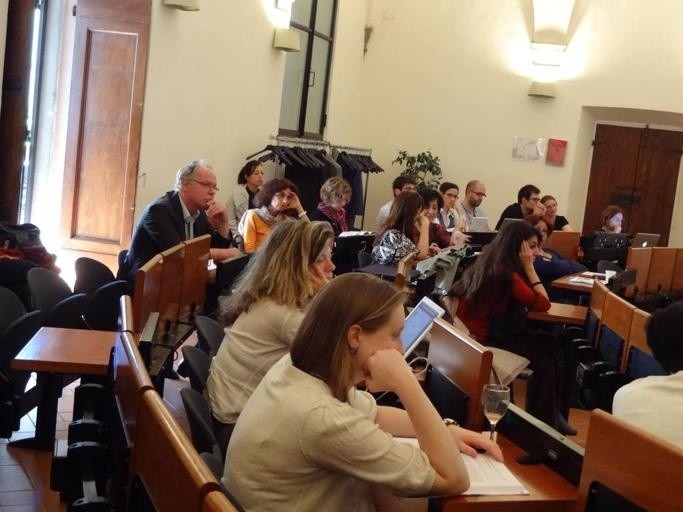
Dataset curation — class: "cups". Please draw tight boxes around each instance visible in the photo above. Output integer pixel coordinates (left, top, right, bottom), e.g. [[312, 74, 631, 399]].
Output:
[[605, 270, 616, 283]]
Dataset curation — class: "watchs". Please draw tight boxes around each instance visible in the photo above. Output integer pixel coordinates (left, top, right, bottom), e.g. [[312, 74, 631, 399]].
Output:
[[444, 418, 460, 426]]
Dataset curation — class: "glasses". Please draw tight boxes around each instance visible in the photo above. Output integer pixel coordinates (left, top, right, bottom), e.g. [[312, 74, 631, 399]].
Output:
[[445, 193, 458, 198], [191, 178, 220, 192], [473, 192, 486, 197]]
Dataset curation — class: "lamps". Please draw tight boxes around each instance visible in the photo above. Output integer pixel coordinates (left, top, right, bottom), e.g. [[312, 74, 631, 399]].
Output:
[[162, 0, 200, 12], [273, 27, 299, 54], [528, 79, 558, 102]]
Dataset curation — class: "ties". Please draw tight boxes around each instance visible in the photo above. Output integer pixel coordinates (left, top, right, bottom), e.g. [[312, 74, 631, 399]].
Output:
[[185, 220, 191, 240]]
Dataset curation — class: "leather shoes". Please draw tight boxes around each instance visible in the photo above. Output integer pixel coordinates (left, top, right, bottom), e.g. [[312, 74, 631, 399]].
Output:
[[557, 416, 576, 436]]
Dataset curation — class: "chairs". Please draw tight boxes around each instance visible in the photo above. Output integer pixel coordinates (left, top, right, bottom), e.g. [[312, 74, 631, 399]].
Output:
[[1, 229, 683, 511]]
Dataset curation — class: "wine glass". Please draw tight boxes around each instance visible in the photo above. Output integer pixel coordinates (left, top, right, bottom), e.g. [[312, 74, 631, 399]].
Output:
[[482, 383, 511, 442]]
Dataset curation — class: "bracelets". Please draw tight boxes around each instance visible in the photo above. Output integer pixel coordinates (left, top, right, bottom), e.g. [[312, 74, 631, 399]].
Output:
[[532, 282, 543, 287], [298, 211, 307, 218]]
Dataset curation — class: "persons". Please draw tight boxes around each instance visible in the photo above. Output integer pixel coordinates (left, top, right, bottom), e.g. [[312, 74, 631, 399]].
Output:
[[495, 184, 546, 230], [126, 160, 244, 302], [307, 176, 360, 250], [540, 195, 572, 231], [433, 182, 465, 232], [221, 271, 504, 510], [0, 227, 40, 313], [583, 205, 624, 273], [371, 190, 441, 268], [524, 215, 586, 304], [447, 220, 578, 436], [612, 301, 682, 449], [208, 217, 336, 466], [237, 178, 309, 259], [225, 159, 265, 252], [456, 179, 492, 232], [410, 189, 470, 254], [376, 175, 416, 231]]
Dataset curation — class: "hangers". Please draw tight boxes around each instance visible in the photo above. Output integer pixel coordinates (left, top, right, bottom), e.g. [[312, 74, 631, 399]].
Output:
[[245, 144, 384, 176]]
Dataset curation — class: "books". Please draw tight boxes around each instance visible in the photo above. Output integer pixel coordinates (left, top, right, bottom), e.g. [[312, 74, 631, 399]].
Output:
[[339, 230, 377, 237], [393, 435, 530, 501], [471, 217, 489, 232]]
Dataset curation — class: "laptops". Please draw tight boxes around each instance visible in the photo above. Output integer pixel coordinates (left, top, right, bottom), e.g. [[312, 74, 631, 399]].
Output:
[[468, 217, 488, 233], [631, 232, 661, 248], [399, 296, 445, 360]]
